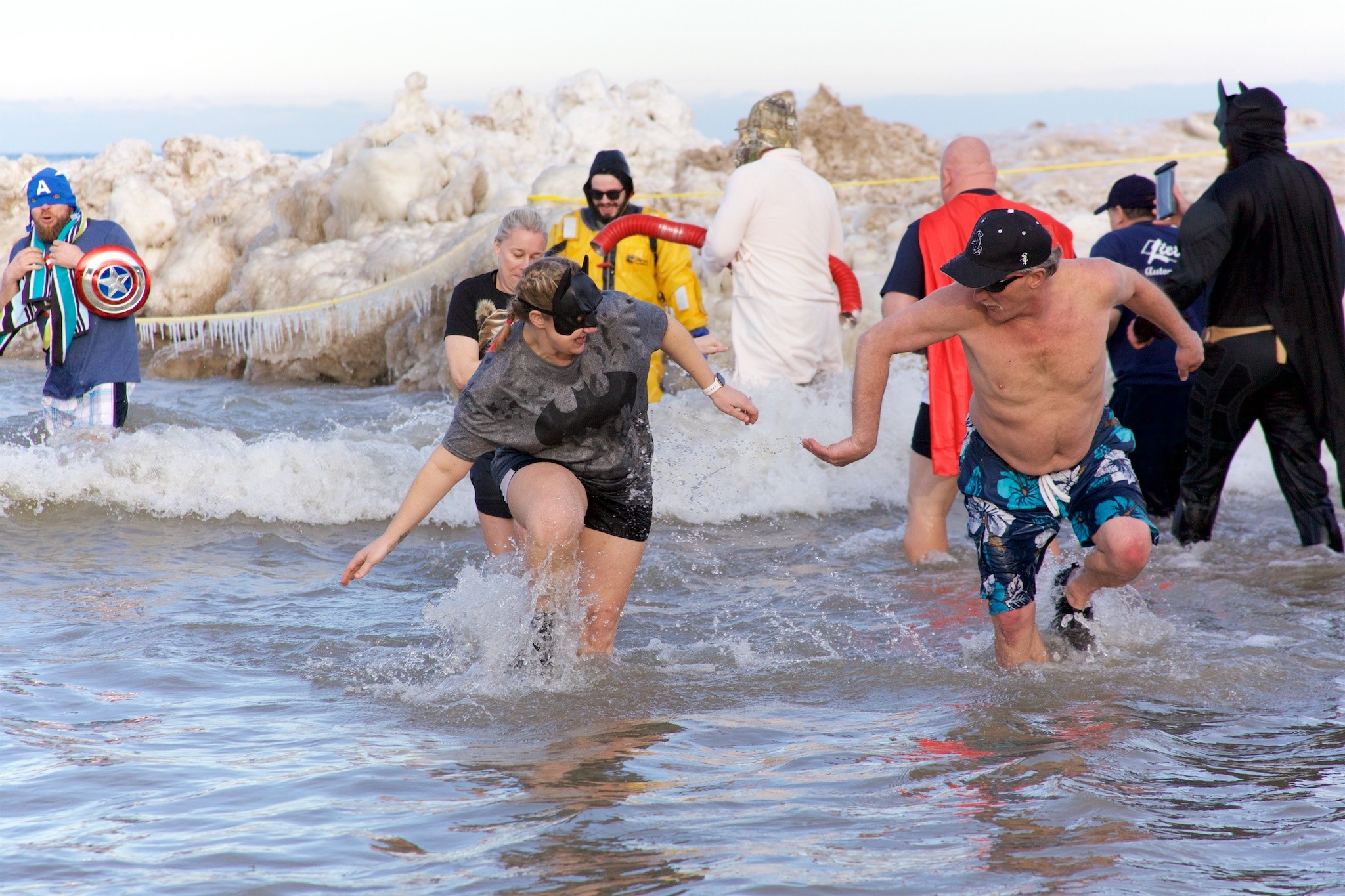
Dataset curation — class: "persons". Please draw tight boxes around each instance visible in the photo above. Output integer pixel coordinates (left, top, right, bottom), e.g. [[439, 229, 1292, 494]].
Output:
[[442, 208, 728, 557], [547, 150, 709, 404], [700, 92, 844, 385], [0, 168, 151, 445], [803, 209, 1206, 667], [881, 137, 1078, 565], [1090, 174, 1209, 519], [1110, 79, 1345, 555], [339, 257, 759, 661]]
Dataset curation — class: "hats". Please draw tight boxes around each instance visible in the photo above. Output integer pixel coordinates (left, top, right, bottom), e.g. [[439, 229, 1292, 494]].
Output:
[[25, 168, 79, 232], [735, 92, 797, 162], [940, 209, 1052, 288], [1094, 175, 1156, 214], [734, 118, 748, 130]]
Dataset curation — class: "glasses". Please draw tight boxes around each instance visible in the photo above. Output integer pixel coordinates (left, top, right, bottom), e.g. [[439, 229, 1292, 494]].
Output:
[[982, 273, 1026, 292], [592, 188, 625, 200]]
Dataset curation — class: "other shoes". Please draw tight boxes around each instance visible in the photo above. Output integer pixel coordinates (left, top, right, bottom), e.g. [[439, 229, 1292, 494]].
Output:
[[529, 606, 558, 669], [1051, 561, 1093, 651]]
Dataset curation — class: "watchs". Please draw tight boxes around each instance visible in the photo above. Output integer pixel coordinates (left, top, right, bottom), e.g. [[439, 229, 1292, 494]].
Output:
[[701, 373, 725, 396]]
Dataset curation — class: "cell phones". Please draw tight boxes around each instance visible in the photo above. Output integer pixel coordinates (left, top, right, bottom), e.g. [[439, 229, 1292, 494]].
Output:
[[1156, 169, 1175, 220]]
